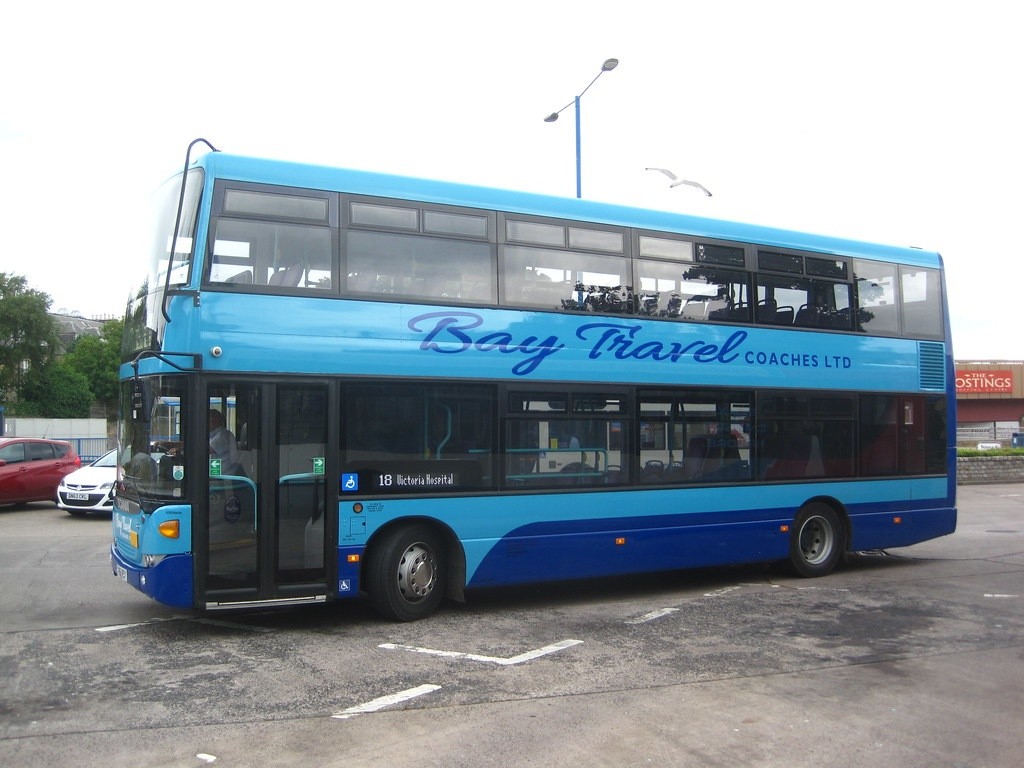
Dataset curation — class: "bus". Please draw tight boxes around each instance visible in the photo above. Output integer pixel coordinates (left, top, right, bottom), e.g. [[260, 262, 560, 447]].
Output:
[[110, 138, 960, 622], [520, 400, 776, 479]]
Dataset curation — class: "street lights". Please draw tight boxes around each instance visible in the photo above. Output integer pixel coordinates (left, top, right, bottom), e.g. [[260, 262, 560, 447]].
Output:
[[544, 56, 619, 305]]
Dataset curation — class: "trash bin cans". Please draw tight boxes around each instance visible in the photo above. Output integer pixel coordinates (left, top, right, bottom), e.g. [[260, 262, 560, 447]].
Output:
[[1012, 433, 1024, 448]]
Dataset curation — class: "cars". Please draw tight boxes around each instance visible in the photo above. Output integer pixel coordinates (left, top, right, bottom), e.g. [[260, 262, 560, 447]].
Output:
[[612, 422, 653, 447], [0, 437, 81, 505], [57, 442, 175, 514]]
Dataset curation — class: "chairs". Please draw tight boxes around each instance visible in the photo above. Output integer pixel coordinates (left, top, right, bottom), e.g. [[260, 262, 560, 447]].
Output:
[[222, 265, 825, 329], [599, 459, 749, 486]]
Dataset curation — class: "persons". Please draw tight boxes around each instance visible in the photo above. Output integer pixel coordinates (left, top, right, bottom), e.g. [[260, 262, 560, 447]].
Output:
[[153, 409, 238, 474]]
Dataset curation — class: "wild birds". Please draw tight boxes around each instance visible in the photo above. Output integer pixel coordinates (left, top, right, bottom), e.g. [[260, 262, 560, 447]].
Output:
[[645, 168, 712, 197]]
